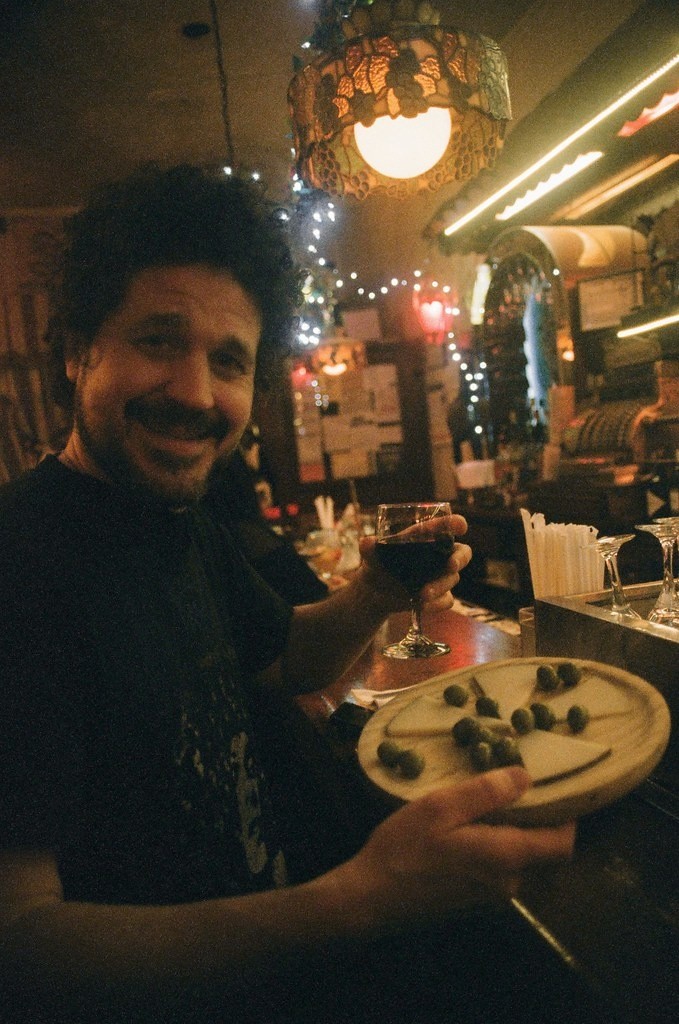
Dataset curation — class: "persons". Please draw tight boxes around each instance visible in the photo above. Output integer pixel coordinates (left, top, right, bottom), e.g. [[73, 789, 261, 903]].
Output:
[[219, 451, 332, 607], [0, 156, 579, 1024]]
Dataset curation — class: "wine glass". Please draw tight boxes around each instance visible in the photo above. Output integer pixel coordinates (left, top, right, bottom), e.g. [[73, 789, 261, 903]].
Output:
[[581, 533, 644, 621], [370, 502, 458, 662], [633, 524, 679, 630], [304, 529, 344, 588]]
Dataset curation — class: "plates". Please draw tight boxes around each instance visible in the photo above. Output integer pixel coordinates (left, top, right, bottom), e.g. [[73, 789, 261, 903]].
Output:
[[356, 656, 671, 823]]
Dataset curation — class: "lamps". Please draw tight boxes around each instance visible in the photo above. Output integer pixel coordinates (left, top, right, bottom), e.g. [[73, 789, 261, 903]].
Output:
[[284, 2, 516, 205]]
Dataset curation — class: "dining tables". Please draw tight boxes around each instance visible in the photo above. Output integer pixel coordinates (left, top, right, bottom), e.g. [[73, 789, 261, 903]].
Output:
[[279, 553, 679, 1024]]
